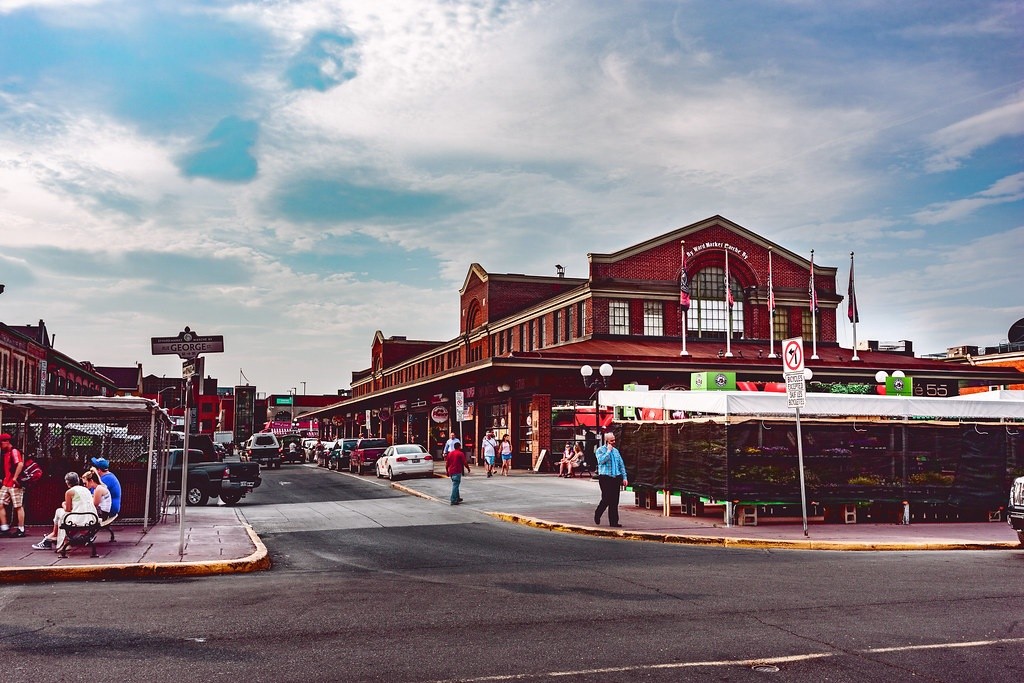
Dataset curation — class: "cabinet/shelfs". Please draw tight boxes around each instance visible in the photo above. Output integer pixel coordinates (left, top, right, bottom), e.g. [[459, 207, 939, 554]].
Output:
[[484, 401, 509, 442], [519, 412, 533, 453]]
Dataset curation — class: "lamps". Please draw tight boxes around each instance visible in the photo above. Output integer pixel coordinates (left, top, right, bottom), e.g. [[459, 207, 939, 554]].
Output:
[[399, 403, 407, 412], [496, 383, 513, 404]]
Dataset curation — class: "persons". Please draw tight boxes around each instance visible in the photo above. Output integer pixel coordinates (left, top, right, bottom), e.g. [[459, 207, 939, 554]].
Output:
[[498, 434, 512, 476], [0, 433, 25, 537], [92, 457, 121, 517], [238, 442, 247, 455], [443, 433, 460, 457], [228, 441, 234, 456], [315, 439, 324, 467], [554, 442, 584, 478], [289, 441, 297, 464], [359, 434, 364, 439], [32, 472, 98, 549], [446, 443, 470, 504], [594, 432, 628, 527], [481, 430, 498, 477], [81, 466, 111, 521], [585, 428, 594, 439]]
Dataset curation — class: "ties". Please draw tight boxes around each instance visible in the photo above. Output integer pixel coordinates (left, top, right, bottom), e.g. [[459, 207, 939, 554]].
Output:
[[610, 451, 616, 477]]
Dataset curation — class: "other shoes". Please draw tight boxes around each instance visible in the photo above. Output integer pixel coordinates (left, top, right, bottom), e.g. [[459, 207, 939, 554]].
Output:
[[493, 470, 497, 474], [0, 528, 9, 537], [564, 474, 571, 477], [610, 523, 622, 528], [12, 527, 25, 538], [458, 497, 463, 501], [451, 501, 459, 505], [594, 513, 600, 525], [45, 532, 57, 540]]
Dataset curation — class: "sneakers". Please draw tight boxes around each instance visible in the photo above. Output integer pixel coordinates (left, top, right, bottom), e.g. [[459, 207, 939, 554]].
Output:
[[32, 539, 52, 549]]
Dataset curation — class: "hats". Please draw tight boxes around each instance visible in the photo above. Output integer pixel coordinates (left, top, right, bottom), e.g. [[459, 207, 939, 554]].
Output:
[[91, 457, 109, 469], [0, 433, 12, 441]]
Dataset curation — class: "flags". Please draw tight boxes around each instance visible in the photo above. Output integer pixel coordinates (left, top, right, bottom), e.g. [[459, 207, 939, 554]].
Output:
[[725, 261, 733, 310], [848, 267, 859, 323], [766, 262, 775, 316], [808, 263, 819, 311], [680, 253, 690, 311]]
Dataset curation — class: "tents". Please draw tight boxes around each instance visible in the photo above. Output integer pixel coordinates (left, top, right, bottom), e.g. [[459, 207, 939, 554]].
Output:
[[599, 389, 1023, 527]]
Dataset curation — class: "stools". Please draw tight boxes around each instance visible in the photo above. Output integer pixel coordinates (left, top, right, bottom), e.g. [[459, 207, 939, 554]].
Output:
[[160, 490, 181, 524]]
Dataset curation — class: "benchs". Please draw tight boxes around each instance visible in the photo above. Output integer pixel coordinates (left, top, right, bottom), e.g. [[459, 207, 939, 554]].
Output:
[[58, 512, 118, 559], [563, 461, 592, 479]]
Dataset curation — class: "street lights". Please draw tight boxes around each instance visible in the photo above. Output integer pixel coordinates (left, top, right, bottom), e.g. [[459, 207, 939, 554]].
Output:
[[158, 386, 177, 405], [580, 362, 614, 448]]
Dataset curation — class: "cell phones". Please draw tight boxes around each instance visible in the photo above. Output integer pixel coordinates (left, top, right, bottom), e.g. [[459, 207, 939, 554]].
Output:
[[606, 441, 610, 444]]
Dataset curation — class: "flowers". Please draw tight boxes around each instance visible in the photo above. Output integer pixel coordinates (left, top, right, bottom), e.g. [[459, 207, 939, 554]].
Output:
[[702, 443, 954, 486]]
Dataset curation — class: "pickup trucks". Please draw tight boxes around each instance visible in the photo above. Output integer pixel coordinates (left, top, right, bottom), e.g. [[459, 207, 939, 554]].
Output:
[[128, 448, 262, 507], [348, 437, 390, 475]]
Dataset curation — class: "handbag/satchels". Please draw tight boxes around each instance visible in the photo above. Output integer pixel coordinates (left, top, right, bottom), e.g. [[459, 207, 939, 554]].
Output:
[[9, 446, 43, 486]]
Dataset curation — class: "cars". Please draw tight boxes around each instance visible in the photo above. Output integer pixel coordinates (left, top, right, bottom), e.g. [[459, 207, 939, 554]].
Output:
[[375, 444, 435, 481], [238, 433, 336, 469], [166, 431, 233, 463]]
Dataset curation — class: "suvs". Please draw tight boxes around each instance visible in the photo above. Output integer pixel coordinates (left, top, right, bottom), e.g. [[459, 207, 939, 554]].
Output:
[[328, 438, 359, 471], [1006, 476, 1024, 547]]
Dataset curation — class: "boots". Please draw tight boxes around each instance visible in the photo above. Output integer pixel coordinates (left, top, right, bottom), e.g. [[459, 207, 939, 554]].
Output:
[[486, 470, 492, 478]]
